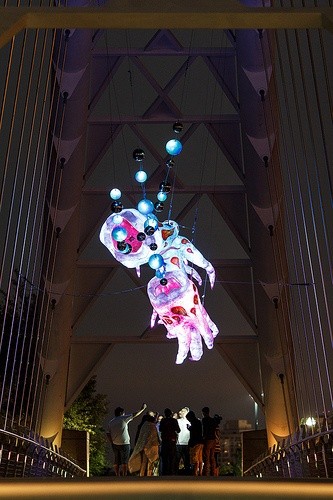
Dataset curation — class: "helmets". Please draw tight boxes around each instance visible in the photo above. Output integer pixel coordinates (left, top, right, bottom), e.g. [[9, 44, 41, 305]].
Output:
[[159, 219, 179, 245]]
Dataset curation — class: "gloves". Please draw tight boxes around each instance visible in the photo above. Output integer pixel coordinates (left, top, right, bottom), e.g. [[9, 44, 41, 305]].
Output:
[[206, 264, 215, 289]]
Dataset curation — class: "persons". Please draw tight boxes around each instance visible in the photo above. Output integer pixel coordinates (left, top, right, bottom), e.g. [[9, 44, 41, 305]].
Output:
[[202, 407, 222, 476], [171, 407, 191, 473], [106, 403, 147, 477], [135, 411, 160, 476], [159, 408, 180, 475], [186, 411, 203, 480]]
